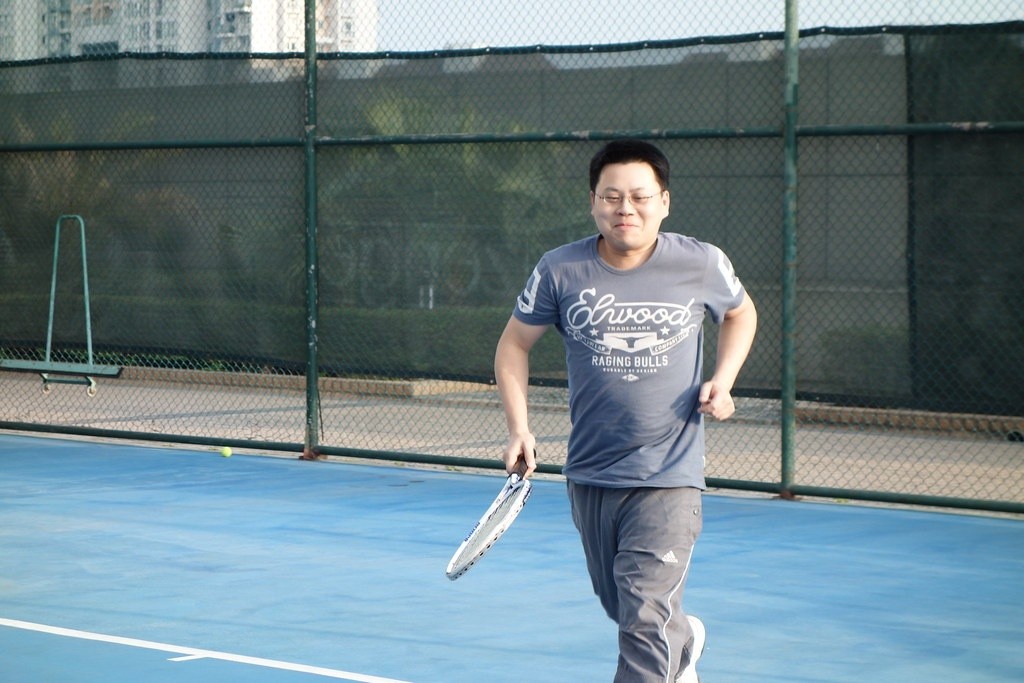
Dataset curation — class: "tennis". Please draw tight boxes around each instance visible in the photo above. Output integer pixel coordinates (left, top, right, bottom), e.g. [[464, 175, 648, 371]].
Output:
[[222, 446, 233, 458]]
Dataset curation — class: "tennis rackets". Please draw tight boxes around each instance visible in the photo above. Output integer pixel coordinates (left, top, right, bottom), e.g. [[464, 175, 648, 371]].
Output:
[[444, 450, 538, 580]]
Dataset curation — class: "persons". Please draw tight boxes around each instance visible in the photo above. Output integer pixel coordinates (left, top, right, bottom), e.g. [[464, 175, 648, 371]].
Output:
[[495, 140, 757, 683]]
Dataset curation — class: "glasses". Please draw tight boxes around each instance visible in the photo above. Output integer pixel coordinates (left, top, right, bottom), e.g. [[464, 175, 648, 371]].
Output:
[[595, 190, 666, 206]]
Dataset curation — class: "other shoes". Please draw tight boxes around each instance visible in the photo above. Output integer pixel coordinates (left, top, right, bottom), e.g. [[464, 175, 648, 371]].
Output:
[[675, 614, 706, 683]]
